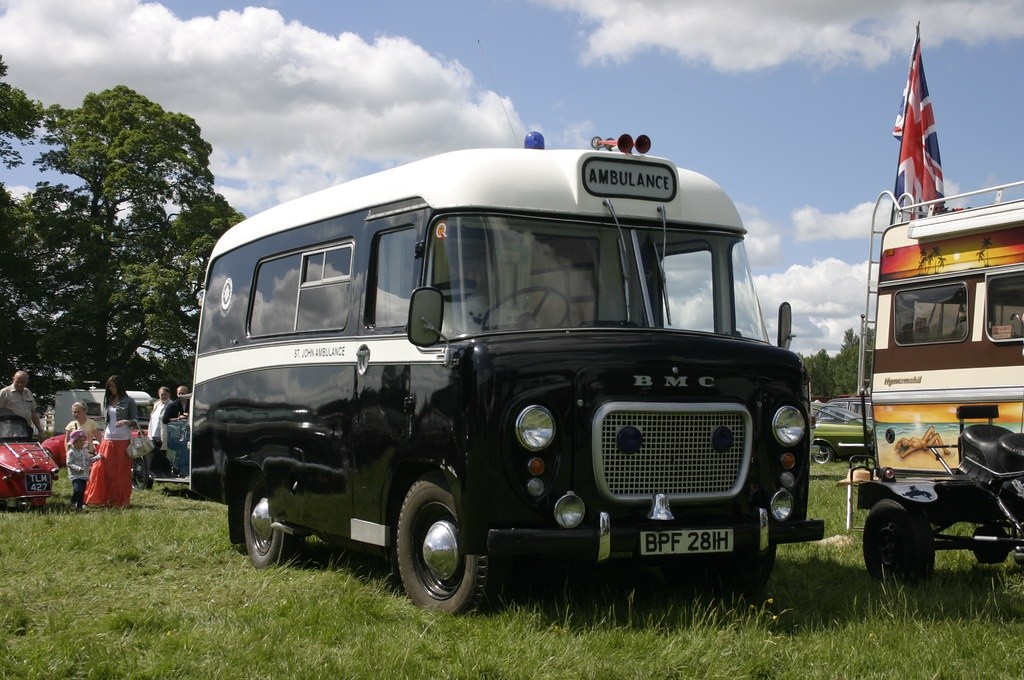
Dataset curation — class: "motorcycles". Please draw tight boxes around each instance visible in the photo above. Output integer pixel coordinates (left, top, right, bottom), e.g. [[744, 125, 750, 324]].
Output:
[[846, 403, 1024, 586]]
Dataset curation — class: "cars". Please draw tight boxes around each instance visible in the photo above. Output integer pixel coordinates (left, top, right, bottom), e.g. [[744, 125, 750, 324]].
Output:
[[41, 428, 149, 468], [811, 397, 874, 464]]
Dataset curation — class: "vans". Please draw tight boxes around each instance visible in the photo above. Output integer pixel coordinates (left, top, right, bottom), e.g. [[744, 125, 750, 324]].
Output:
[[188, 131, 825, 621], [53, 381, 154, 444]]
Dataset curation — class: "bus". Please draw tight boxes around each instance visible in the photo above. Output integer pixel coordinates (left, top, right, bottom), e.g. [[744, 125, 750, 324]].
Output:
[[855, 180, 1024, 501]]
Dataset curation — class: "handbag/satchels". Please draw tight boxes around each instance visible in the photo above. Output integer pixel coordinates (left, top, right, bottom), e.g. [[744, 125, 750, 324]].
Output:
[[126, 419, 154, 460]]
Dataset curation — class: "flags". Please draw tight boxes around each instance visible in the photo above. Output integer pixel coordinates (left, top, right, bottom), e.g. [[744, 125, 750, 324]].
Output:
[[891, 26, 946, 222]]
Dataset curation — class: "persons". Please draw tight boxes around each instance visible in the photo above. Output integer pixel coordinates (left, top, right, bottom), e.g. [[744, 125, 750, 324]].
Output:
[[0, 371, 46, 439], [148, 385, 190, 478], [65, 430, 103, 516], [82, 375, 138, 512], [65, 400, 102, 471]]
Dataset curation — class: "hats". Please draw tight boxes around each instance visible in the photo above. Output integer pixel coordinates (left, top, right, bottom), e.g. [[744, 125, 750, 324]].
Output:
[[836, 469, 870, 486], [68, 430, 89, 445]]
